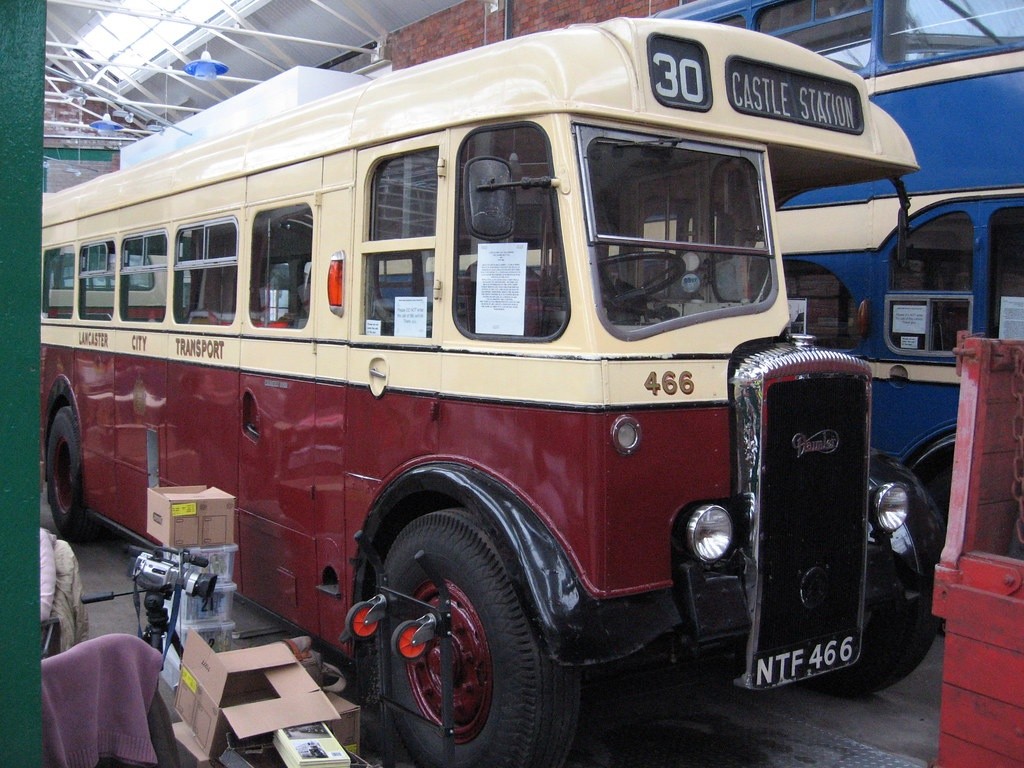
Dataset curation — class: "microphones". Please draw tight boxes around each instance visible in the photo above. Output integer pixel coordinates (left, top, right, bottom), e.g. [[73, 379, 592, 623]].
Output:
[[158, 547, 209, 568]]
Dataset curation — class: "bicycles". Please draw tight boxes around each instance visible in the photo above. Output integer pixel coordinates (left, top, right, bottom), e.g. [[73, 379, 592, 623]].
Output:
[[39, 527, 367, 768]]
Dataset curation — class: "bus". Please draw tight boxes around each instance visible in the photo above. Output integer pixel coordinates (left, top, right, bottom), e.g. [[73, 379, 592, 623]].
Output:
[[649, 0, 1024, 540], [41, 18, 941, 768]]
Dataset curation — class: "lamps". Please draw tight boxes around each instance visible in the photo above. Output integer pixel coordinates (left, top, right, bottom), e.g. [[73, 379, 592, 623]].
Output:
[[182, 28, 229, 81], [88, 70, 124, 130]]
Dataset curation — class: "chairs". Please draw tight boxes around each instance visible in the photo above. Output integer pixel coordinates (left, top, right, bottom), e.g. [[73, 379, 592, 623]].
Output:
[[43, 537, 78, 654], [43, 632, 179, 768]]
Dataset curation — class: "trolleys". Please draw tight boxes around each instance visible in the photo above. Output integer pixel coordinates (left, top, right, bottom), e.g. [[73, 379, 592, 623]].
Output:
[[344, 527, 453, 768]]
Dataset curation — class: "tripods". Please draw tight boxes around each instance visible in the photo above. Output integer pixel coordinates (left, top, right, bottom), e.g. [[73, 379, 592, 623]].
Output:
[[80, 587, 185, 670]]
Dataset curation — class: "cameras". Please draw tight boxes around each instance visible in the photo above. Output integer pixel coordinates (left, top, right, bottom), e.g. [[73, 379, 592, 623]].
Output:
[[132, 552, 217, 599]]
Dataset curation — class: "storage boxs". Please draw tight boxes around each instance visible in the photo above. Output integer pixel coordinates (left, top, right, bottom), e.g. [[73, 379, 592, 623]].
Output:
[[144, 484, 375, 768]]
